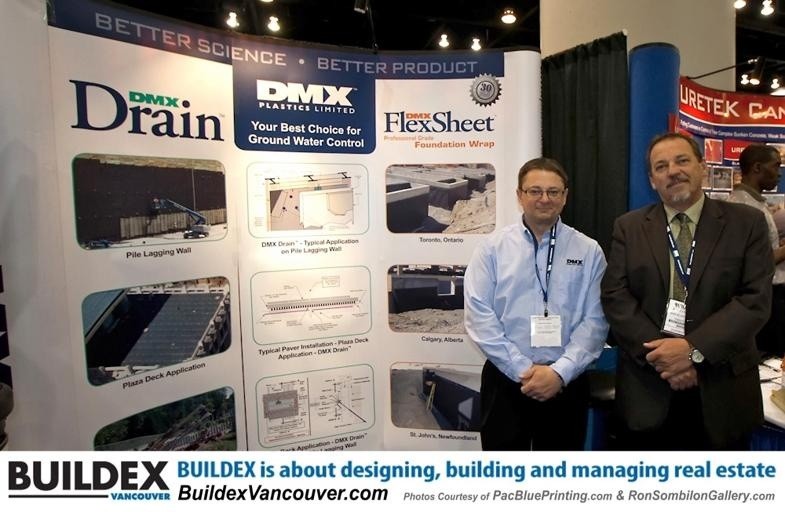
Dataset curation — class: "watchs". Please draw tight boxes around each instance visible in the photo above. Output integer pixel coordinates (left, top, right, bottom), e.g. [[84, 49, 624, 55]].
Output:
[[688, 342, 706, 371]]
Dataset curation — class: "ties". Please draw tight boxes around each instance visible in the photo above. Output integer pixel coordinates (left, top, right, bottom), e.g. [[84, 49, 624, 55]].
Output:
[[673, 213, 693, 302]]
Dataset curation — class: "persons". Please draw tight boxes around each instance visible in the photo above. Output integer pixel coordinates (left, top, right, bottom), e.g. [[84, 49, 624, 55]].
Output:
[[463, 156, 613, 450], [600, 131, 776, 449], [723, 140, 784, 361]]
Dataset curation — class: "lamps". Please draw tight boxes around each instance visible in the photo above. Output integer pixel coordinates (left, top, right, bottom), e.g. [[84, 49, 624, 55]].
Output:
[[749, 57, 764, 85]]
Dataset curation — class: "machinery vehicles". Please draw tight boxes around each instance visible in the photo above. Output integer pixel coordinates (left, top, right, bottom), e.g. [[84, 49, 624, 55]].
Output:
[[147, 198, 211, 238]]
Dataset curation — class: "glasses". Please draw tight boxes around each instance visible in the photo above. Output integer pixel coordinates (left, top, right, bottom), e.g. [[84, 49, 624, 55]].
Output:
[[521, 188, 564, 197]]
